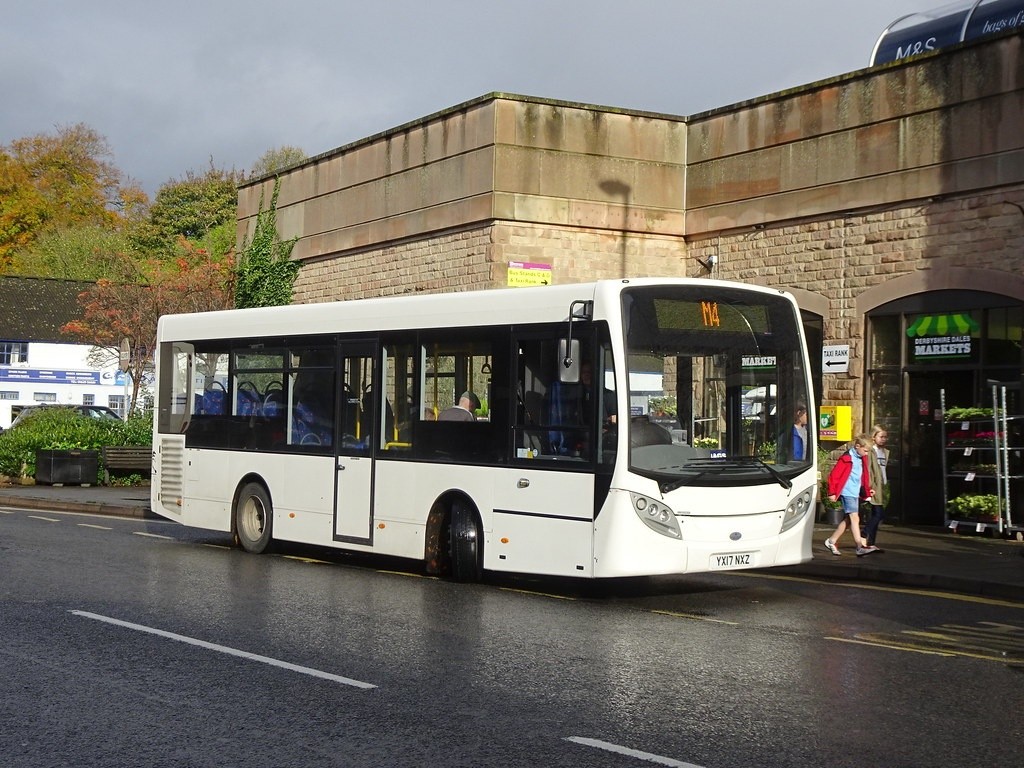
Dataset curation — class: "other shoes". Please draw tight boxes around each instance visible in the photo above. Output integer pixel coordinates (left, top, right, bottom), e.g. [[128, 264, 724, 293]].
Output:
[[867, 545, 885, 553]]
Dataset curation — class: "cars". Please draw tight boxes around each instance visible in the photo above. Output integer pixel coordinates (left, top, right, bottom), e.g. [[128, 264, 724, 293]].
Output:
[[9, 402, 124, 428]]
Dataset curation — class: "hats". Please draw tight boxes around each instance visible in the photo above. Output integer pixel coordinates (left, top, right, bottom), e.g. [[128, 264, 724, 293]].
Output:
[[461, 391, 481, 409]]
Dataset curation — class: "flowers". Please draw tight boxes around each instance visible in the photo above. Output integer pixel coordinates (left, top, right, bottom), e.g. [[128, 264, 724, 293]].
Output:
[[647, 394, 674, 410], [694, 437, 718, 449], [948, 430, 1004, 439]]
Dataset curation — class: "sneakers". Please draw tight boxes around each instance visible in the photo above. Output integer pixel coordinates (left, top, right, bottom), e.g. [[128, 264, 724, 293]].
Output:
[[856, 545, 876, 557], [823, 537, 842, 556]]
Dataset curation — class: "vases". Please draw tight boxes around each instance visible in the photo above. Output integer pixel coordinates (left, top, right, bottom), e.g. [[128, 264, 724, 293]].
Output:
[[949, 438, 1004, 447], [651, 407, 665, 417], [858, 511, 869, 525]]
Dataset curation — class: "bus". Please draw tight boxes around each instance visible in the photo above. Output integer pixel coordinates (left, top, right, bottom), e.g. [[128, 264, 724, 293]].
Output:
[[149, 274, 832, 588]]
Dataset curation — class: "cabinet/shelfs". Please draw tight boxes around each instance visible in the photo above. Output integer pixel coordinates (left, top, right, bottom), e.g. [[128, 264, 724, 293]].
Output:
[[1000, 386, 1024, 541], [940, 385, 1016, 537]]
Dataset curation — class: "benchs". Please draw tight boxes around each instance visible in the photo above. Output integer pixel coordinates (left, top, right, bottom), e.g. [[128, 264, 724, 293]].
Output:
[[102, 443, 152, 483]]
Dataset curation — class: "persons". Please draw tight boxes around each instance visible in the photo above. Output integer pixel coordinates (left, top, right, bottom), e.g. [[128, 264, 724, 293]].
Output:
[[794, 399, 807, 461], [824, 433, 876, 557], [433, 391, 482, 422], [860, 423, 890, 553]]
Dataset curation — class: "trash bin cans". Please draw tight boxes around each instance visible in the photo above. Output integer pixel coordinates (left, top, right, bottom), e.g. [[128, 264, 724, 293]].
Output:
[[34, 449, 98, 487]]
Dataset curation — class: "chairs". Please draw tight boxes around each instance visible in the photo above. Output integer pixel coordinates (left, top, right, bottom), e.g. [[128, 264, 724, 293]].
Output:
[[342, 435, 360, 448], [364, 384, 394, 422], [291, 416, 300, 445], [237, 381, 261, 415], [344, 383, 356, 412], [296, 396, 322, 433], [262, 393, 282, 416], [264, 381, 283, 403], [300, 434, 322, 447], [202, 381, 229, 415]]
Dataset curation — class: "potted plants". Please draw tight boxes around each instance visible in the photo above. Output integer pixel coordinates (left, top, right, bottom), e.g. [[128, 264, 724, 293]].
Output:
[[820, 481, 845, 524], [944, 406, 1004, 422], [947, 494, 1006, 521]]
